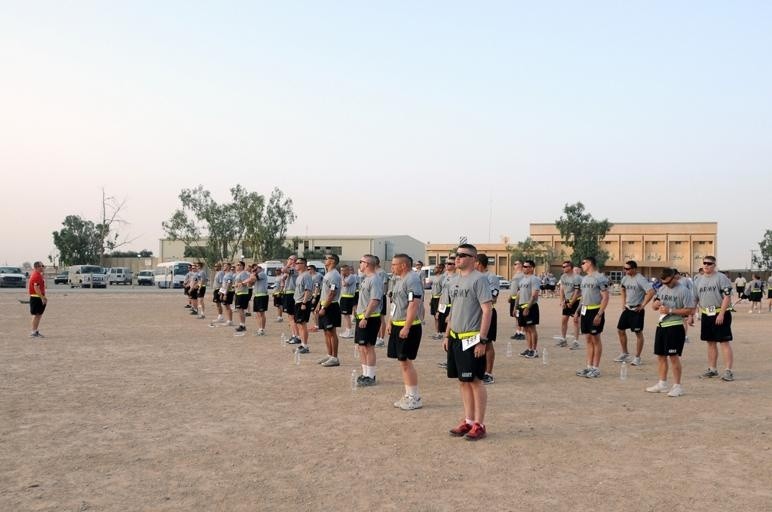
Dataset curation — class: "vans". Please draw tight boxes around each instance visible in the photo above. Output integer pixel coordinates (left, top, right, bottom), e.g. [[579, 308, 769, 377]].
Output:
[[256, 257, 285, 289], [307, 260, 327, 277], [55, 261, 193, 290]]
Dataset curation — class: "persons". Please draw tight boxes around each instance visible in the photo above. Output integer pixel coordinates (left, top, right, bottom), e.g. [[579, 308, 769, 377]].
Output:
[[28, 261, 48, 337]]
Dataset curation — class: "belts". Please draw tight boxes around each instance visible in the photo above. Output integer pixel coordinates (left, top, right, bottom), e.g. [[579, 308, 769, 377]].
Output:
[[511, 295, 683, 328]]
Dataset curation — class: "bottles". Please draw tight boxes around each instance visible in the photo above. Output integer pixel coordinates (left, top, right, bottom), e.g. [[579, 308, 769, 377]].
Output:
[[351, 368, 358, 391], [619, 362, 628, 380], [507, 342, 513, 358], [542, 346, 549, 366], [207, 316, 246, 339], [281, 333, 301, 365], [353, 344, 359, 359]]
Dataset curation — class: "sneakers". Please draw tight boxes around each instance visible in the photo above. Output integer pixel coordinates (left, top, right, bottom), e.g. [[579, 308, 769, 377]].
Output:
[[697, 368, 719, 379], [645, 380, 668, 394], [285, 334, 310, 354], [612, 353, 631, 362], [510, 332, 526, 341], [720, 368, 736, 382], [520, 349, 538, 358], [569, 340, 580, 350], [630, 356, 643, 366], [28, 331, 47, 339], [355, 374, 376, 387], [184, 304, 251, 332], [339, 331, 353, 338], [667, 384, 684, 398], [317, 354, 340, 366], [684, 307, 772, 343], [556, 338, 568, 347], [394, 393, 425, 412], [449, 420, 489, 441], [375, 336, 385, 347], [576, 367, 603, 379], [481, 372, 495, 385]]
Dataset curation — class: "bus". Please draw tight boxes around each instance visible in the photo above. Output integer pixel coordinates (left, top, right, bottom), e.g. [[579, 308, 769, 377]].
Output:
[[412, 265, 436, 289]]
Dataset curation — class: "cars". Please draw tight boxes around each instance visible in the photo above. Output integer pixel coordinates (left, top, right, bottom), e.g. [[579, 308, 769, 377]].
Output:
[[0, 266, 29, 287], [496, 275, 510, 289]]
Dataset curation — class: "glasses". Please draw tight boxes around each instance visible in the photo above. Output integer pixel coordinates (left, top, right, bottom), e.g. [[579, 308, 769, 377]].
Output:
[[703, 261, 715, 265], [661, 277, 674, 285], [512, 260, 590, 270], [37, 264, 45, 268], [624, 267, 634, 271], [187, 257, 456, 273], [454, 252, 474, 259]]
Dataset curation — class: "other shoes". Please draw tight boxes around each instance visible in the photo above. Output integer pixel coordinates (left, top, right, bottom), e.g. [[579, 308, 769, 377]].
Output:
[[437, 361, 448, 369], [308, 326, 318, 331], [432, 334, 443, 340], [274, 317, 283, 322], [254, 331, 264, 337]]
